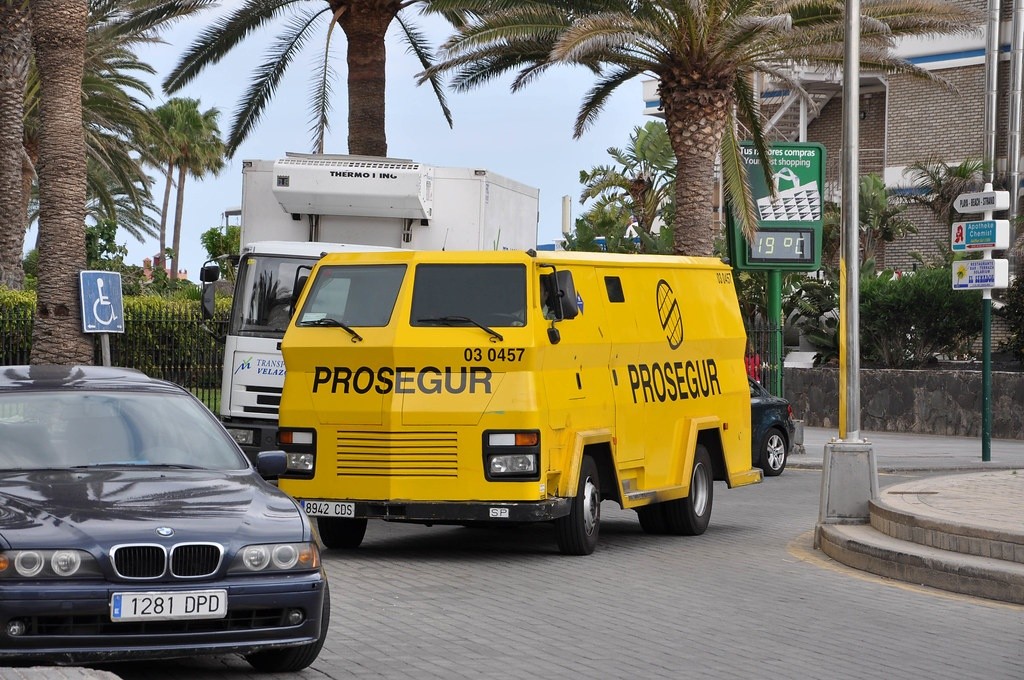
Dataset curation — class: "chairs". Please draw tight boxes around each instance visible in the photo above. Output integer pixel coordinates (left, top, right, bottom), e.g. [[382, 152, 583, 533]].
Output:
[[267, 304, 291, 325], [65, 416, 142, 463]]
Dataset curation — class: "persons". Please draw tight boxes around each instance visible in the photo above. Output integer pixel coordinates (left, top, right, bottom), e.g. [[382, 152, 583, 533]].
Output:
[[745, 337, 761, 393], [513, 276, 556, 326]]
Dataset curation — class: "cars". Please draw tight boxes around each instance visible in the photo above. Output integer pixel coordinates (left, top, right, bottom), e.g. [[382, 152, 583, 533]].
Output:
[[0, 365, 330, 671]]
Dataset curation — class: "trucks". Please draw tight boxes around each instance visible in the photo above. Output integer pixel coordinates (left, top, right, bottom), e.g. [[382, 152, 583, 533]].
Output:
[[275, 248, 765, 556], [198, 151, 539, 488]]
